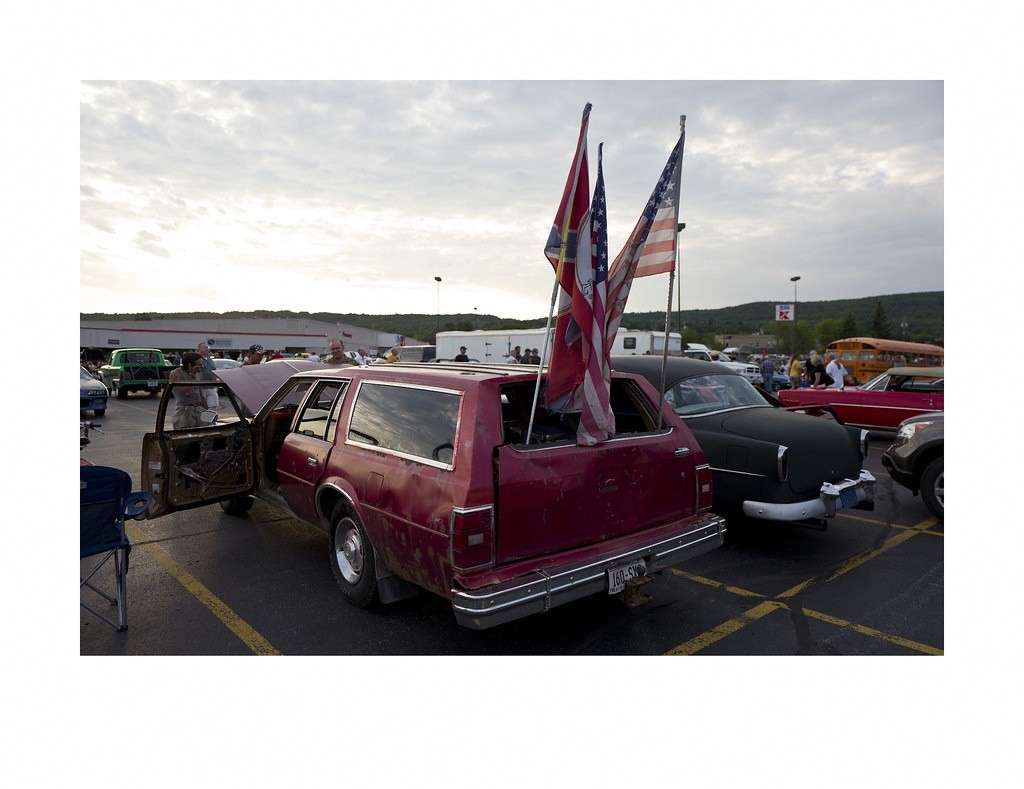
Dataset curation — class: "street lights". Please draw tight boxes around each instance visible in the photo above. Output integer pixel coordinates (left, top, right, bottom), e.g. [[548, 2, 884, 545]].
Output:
[[434, 276, 442, 332], [671, 221, 686, 335], [791, 275, 801, 359]]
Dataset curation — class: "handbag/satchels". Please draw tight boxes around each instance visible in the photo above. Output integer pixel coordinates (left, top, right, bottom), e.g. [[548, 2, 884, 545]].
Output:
[[824, 373, 835, 386]]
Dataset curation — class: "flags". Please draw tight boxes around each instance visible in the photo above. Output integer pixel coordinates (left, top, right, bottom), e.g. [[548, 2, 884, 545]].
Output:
[[542, 104, 685, 446]]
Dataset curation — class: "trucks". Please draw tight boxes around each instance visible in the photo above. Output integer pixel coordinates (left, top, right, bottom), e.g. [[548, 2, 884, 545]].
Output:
[[687, 342, 765, 387]]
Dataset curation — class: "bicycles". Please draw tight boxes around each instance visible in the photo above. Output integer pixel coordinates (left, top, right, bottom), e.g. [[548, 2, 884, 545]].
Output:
[[80, 421, 105, 460]]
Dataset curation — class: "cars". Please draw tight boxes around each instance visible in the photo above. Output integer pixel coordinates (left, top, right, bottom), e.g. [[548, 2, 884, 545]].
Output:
[[880, 409, 944, 528], [210, 359, 242, 396], [138, 356, 728, 632], [603, 356, 880, 543], [773, 367, 944, 433], [80, 366, 110, 417], [896, 378, 944, 392]]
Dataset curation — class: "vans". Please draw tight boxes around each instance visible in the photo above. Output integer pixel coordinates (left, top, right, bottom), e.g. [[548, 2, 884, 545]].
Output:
[[382, 344, 437, 363]]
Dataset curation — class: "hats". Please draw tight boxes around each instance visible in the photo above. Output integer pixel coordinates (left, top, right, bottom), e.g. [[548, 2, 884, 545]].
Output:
[[250, 344, 267, 355], [460, 346, 467, 349], [525, 348, 532, 352]]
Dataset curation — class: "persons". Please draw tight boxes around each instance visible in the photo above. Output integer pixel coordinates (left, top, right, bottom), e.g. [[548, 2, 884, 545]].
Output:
[[455, 346, 469, 362], [507, 346, 541, 365], [171, 350, 230, 360], [324, 338, 359, 366], [296, 352, 303, 358], [239, 344, 267, 417], [632, 349, 689, 359], [751, 350, 847, 393], [272, 350, 283, 358], [169, 353, 208, 428], [198, 341, 219, 412], [309, 351, 320, 362], [354, 349, 366, 365], [388, 348, 400, 362]]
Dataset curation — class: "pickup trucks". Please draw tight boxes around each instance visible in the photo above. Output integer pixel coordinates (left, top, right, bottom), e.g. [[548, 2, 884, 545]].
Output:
[[97, 347, 184, 400]]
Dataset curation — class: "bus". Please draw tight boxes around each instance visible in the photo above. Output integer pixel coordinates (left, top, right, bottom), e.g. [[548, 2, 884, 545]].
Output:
[[822, 336, 944, 384]]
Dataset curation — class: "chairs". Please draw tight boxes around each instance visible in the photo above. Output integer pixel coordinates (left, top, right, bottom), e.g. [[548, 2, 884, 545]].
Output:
[[80, 463, 153, 633]]
